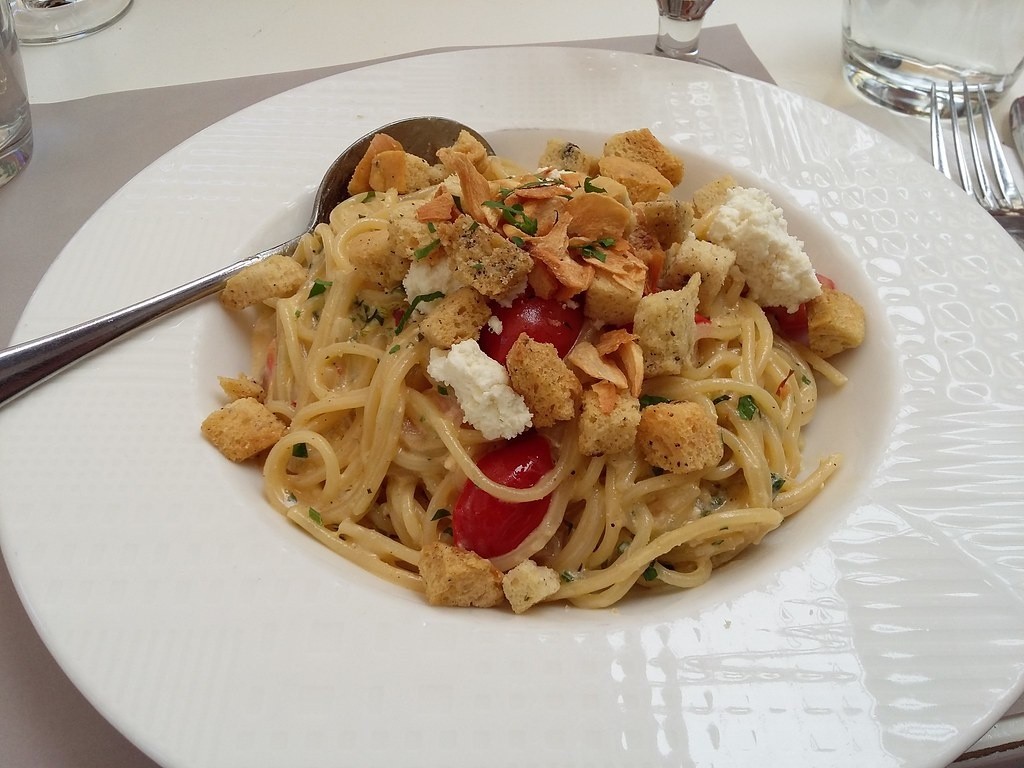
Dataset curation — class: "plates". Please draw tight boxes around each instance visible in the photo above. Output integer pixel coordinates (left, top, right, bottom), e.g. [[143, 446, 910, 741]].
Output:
[[0, 44, 1024, 768]]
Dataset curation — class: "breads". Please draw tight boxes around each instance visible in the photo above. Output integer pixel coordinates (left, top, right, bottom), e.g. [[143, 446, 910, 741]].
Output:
[[198, 127, 866, 614]]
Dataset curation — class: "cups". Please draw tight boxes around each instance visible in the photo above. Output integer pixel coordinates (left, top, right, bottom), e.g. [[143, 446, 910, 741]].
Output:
[[840, 0, 1024, 123], [0, 0, 33, 189]]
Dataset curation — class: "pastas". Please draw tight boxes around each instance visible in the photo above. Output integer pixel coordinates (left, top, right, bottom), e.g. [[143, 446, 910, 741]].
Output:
[[259, 188, 851, 608]]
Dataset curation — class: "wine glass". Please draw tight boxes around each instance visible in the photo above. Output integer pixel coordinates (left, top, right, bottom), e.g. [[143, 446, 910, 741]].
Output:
[[9, 0, 133, 46], [640, 0, 735, 73]]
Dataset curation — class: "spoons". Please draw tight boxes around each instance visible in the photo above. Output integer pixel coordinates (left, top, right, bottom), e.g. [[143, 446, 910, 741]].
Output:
[[0, 117, 497, 410]]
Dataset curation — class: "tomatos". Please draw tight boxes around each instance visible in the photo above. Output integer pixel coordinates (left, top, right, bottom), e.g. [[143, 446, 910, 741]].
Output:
[[454, 431, 559, 558], [481, 292, 585, 365]]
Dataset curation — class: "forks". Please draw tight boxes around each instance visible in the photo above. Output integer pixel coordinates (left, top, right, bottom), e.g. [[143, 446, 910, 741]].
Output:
[[930, 79, 1024, 252]]
